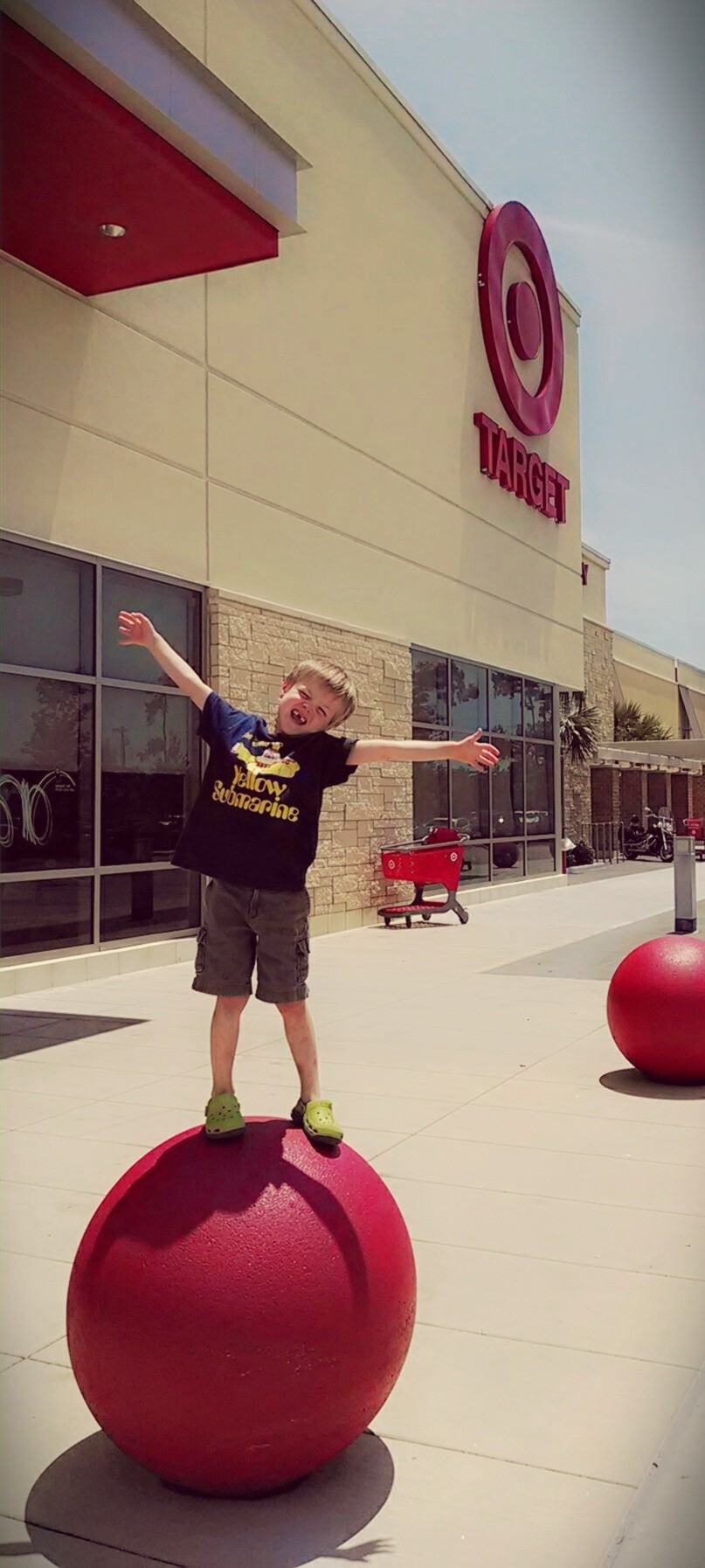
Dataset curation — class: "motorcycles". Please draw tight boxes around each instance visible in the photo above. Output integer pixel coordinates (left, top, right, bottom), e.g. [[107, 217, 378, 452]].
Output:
[[617, 808, 675, 862]]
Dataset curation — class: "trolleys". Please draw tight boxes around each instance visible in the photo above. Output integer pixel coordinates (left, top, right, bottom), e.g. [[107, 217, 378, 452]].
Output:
[[376, 829, 469, 926]]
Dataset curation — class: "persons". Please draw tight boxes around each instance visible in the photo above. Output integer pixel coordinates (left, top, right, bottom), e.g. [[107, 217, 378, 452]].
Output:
[[118, 610, 501, 1145]]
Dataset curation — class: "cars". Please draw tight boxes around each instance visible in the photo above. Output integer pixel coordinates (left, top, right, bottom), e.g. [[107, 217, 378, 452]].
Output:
[[414, 809, 550, 845]]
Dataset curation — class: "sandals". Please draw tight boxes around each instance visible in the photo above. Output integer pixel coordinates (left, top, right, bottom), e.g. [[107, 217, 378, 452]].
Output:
[[205, 1093, 246, 1138], [291, 1097, 344, 1145]]
